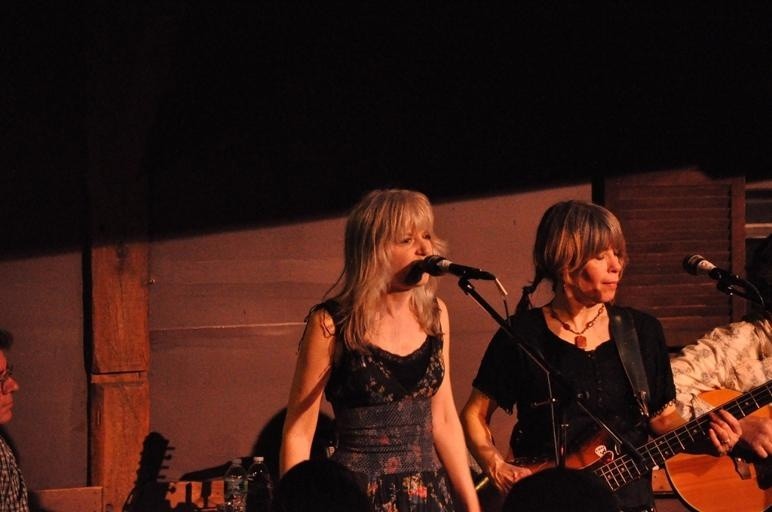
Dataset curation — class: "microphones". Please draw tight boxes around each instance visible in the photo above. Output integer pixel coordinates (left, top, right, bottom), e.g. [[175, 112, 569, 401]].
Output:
[[424, 254, 495, 281], [684, 254, 741, 283]]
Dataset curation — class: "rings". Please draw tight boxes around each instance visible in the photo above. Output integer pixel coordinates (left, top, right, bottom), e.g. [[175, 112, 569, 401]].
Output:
[[723, 438, 731, 446]]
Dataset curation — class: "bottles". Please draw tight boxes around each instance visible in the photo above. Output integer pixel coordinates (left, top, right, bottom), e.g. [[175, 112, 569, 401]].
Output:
[[223, 455, 276, 512]]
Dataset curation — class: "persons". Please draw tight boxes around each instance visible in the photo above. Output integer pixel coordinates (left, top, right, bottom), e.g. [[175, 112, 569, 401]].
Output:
[[650, 319, 772, 457], [460, 202, 743, 511], [501, 467, 618, 512], [282, 187, 482, 512], [0, 330, 31, 511], [272, 459, 375, 512]]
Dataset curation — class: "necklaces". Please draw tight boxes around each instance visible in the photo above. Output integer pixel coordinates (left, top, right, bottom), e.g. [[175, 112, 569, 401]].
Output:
[[551, 302, 606, 349]]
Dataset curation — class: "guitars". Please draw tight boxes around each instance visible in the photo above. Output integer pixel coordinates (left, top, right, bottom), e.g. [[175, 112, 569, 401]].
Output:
[[470, 381, 772, 512], [663, 389, 772, 512]]
